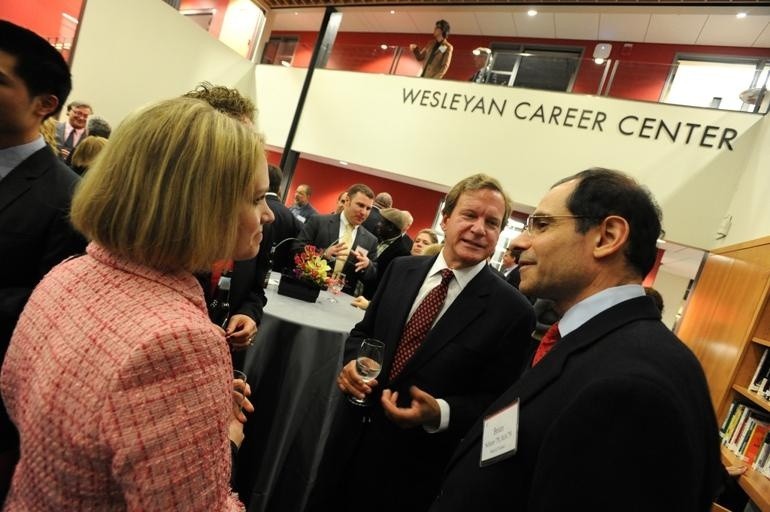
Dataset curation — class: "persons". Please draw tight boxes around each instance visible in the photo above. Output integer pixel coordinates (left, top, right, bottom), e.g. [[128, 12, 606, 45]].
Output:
[[322, 175, 537, 511], [426, 169, 723, 512], [0, 20, 82, 512], [1, 97, 276, 512], [468, 47, 497, 83], [410, 20, 454, 79]]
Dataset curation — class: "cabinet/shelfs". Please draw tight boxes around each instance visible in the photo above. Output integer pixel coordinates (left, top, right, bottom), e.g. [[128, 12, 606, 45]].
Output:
[[711, 290, 770, 511], [675, 238, 770, 411]]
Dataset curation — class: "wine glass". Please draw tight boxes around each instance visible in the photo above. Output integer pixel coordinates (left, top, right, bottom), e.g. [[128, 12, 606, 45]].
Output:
[[347, 337, 386, 407], [326, 271, 346, 304]]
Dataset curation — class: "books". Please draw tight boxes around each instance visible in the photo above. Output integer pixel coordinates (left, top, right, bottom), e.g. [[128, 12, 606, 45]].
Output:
[[719, 347, 770, 479]]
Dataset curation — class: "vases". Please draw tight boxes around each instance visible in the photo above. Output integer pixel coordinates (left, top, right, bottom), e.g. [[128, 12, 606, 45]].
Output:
[[278, 271, 321, 304]]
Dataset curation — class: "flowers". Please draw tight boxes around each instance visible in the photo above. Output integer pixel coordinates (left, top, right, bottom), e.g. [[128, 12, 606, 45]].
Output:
[[293, 243, 340, 291]]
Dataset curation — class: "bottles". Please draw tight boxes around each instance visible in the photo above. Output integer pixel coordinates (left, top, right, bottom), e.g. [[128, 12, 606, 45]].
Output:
[[263, 242, 276, 288], [206, 268, 234, 331]]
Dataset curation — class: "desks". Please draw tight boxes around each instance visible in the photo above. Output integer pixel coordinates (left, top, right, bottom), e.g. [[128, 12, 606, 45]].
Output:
[[227, 266, 368, 510]]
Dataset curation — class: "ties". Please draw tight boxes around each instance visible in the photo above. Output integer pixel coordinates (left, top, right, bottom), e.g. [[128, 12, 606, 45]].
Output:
[[531, 320, 560, 367], [331, 223, 354, 280], [64, 130, 74, 148], [388, 269, 454, 379]]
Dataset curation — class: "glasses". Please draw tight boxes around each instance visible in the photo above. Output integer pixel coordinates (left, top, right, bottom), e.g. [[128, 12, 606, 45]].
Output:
[[522, 214, 585, 236]]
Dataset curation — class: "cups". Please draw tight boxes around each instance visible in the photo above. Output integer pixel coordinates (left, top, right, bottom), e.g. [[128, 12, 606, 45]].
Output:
[[230, 368, 247, 423], [64, 146, 72, 160]]
